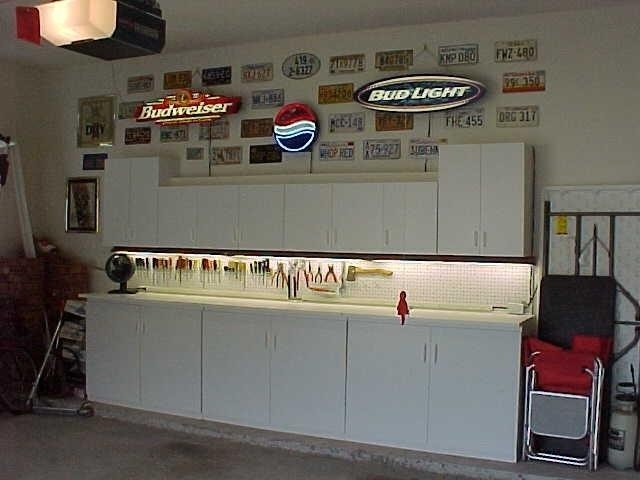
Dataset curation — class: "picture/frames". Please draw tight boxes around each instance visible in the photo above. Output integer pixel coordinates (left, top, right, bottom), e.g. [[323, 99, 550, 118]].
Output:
[[76, 96, 117, 149], [65, 177, 99, 233]]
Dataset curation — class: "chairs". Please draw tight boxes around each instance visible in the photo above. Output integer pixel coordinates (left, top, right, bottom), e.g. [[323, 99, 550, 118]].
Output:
[[522, 349, 606, 473]]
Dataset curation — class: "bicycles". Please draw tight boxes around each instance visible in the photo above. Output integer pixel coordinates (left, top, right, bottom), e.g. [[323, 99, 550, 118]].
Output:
[[0, 338, 42, 416]]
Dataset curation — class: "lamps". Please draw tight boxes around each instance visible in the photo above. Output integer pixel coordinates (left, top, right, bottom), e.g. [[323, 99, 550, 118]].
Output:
[[35, 0, 166, 61]]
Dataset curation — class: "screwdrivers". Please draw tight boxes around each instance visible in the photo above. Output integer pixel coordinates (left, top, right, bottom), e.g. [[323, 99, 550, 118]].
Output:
[[136, 255, 269, 289]]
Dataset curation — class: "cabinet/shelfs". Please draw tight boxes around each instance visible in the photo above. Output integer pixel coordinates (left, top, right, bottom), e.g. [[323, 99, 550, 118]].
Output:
[[437, 143, 535, 259], [203, 307, 345, 440], [87, 298, 202, 421], [157, 186, 196, 254], [103, 155, 158, 250], [282, 183, 383, 259], [345, 316, 523, 465], [196, 184, 283, 256], [383, 182, 437, 260]]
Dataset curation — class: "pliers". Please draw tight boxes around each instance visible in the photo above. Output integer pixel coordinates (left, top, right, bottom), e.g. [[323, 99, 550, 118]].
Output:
[[271, 262, 337, 298]]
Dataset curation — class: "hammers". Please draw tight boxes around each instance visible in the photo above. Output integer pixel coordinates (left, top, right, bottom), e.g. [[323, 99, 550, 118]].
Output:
[[347, 266, 393, 281]]
